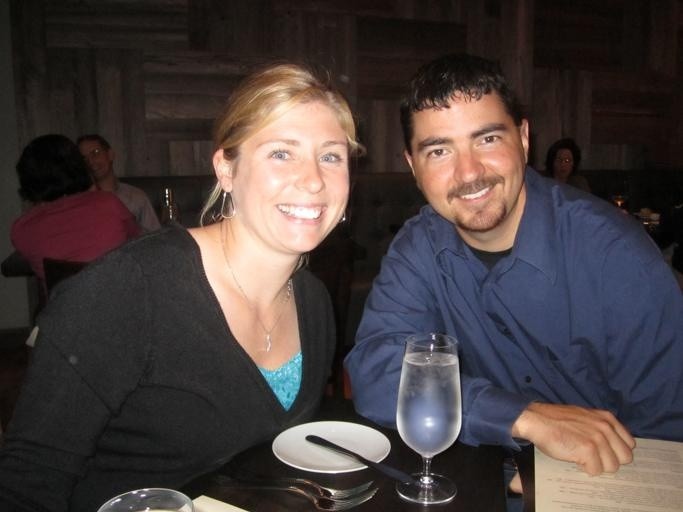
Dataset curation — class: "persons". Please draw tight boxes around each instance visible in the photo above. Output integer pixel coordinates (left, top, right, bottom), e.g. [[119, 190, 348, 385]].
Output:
[[75, 133, 162, 235], [11, 135, 148, 293], [341, 52, 683, 476], [544, 137, 592, 196], [1, 63, 365, 512]]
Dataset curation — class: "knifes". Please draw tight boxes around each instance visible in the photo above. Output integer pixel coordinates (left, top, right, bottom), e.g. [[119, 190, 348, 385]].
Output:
[[304, 434, 419, 489]]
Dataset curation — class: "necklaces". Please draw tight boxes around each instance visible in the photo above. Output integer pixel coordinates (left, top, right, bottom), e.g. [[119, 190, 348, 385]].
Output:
[[219, 228, 292, 353]]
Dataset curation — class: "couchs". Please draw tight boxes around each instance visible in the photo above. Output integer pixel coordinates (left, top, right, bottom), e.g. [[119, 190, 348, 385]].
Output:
[[117, 175, 351, 227], [343, 169, 683, 348]]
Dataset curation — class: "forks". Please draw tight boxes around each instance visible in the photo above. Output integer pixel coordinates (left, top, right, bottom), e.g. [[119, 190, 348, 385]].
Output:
[[235, 475, 379, 510]]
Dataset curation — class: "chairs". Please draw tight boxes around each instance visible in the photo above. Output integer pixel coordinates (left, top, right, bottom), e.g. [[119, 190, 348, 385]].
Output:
[[43, 257, 90, 301]]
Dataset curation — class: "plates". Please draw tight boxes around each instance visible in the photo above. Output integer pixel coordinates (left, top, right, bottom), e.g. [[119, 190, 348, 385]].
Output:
[[270, 420, 392, 477]]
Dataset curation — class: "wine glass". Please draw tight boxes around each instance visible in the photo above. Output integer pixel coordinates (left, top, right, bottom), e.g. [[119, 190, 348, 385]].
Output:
[[395, 332, 464, 504], [97, 487, 194, 512]]
